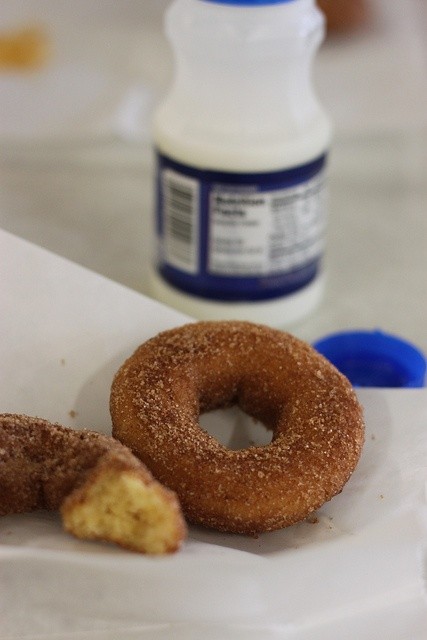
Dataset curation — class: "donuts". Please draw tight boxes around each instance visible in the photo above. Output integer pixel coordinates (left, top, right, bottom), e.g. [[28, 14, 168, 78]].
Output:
[[1, 412, 190, 555], [108, 320, 367, 536]]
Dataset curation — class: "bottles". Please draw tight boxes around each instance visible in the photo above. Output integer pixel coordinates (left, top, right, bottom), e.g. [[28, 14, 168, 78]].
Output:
[[144, 0, 338, 335]]
